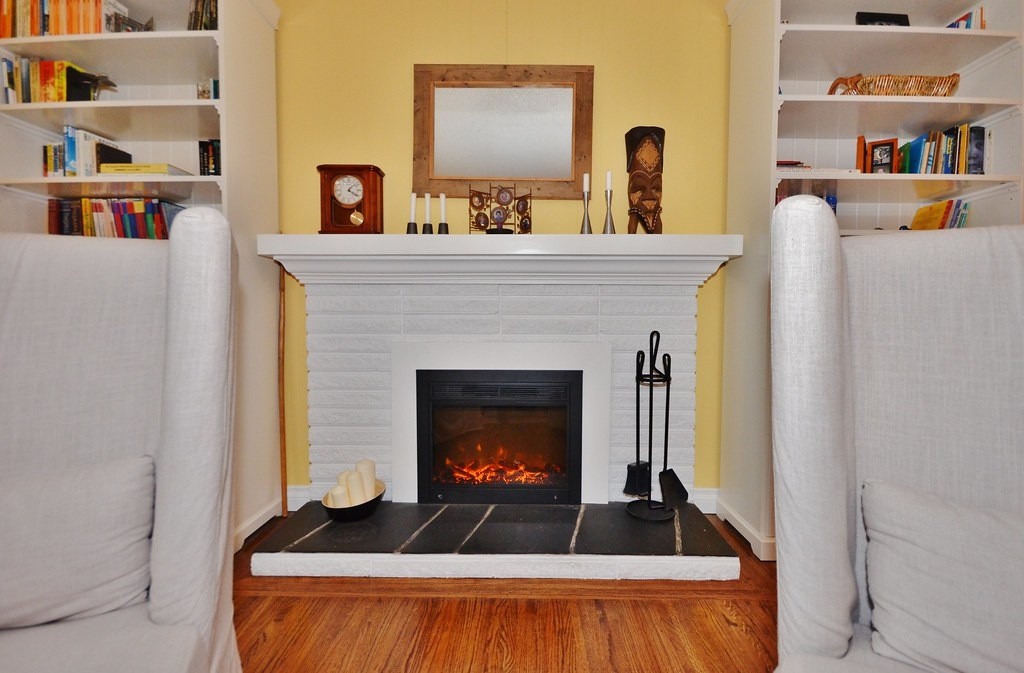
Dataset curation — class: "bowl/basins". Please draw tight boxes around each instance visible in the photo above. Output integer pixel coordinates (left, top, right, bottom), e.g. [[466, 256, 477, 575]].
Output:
[[321, 479, 386, 522]]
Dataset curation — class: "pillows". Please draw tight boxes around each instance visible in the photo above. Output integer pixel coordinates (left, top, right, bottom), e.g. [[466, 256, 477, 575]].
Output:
[[0, 456, 157, 629], [860, 483, 1024, 673]]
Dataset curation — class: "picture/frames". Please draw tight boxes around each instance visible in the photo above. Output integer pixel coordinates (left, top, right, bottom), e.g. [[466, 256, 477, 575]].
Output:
[[866, 138, 898, 174]]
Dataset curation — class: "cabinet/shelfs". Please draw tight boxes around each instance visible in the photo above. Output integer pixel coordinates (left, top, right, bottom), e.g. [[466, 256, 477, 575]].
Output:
[[716, 0, 1024, 564], [0, 0, 286, 555]]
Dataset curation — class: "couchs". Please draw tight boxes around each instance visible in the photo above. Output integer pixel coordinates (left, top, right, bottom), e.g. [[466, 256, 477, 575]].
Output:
[[0, 205, 247, 673], [768, 192, 1024, 673]]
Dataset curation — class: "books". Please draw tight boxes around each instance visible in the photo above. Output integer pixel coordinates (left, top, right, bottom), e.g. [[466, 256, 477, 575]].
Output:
[[775, 123, 986, 229], [0, 0, 222, 240], [946, 7, 986, 30]]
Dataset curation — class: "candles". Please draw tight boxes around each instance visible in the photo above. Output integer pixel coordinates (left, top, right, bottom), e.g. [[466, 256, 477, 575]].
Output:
[[338, 470, 350, 485], [605, 169, 613, 190], [410, 191, 417, 223], [356, 457, 376, 500], [332, 485, 351, 508], [425, 191, 431, 224], [439, 191, 446, 224], [346, 470, 367, 507], [328, 488, 335, 508], [583, 171, 590, 192]]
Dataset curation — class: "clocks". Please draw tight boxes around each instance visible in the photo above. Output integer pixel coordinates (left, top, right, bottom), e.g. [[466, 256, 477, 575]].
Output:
[[316, 164, 386, 234]]
